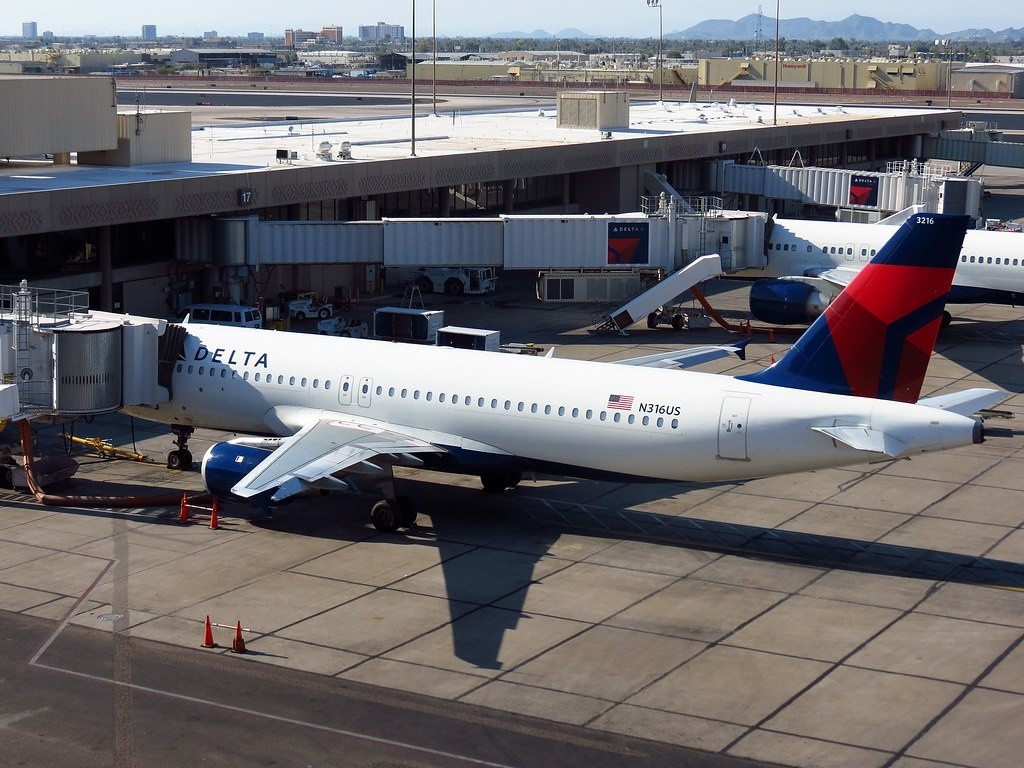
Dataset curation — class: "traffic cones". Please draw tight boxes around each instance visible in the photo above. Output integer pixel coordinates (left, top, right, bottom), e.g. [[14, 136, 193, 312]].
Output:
[[200, 615, 217, 648], [207, 503, 222, 530], [230, 620, 247, 653], [178, 492, 191, 518], [176, 500, 189, 524]]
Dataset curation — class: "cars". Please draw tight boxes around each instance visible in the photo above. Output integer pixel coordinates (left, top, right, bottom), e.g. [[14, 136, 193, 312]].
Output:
[[166, 304, 263, 329]]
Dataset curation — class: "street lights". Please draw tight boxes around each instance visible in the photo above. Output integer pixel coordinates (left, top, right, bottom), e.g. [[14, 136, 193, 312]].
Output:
[[942, 40, 952, 109], [647, 0, 663, 105]]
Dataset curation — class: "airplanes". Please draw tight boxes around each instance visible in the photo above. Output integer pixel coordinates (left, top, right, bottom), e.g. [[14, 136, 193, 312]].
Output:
[[646, 214, 1024, 329], [116, 212, 1008, 534]]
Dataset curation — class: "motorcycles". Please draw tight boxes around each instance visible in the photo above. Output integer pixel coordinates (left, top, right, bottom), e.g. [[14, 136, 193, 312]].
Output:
[[317, 316, 368, 338], [288, 298, 333, 320]]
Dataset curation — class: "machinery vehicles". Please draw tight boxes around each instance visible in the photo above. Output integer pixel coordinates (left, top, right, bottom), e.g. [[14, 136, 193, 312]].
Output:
[[414, 267, 499, 295]]
